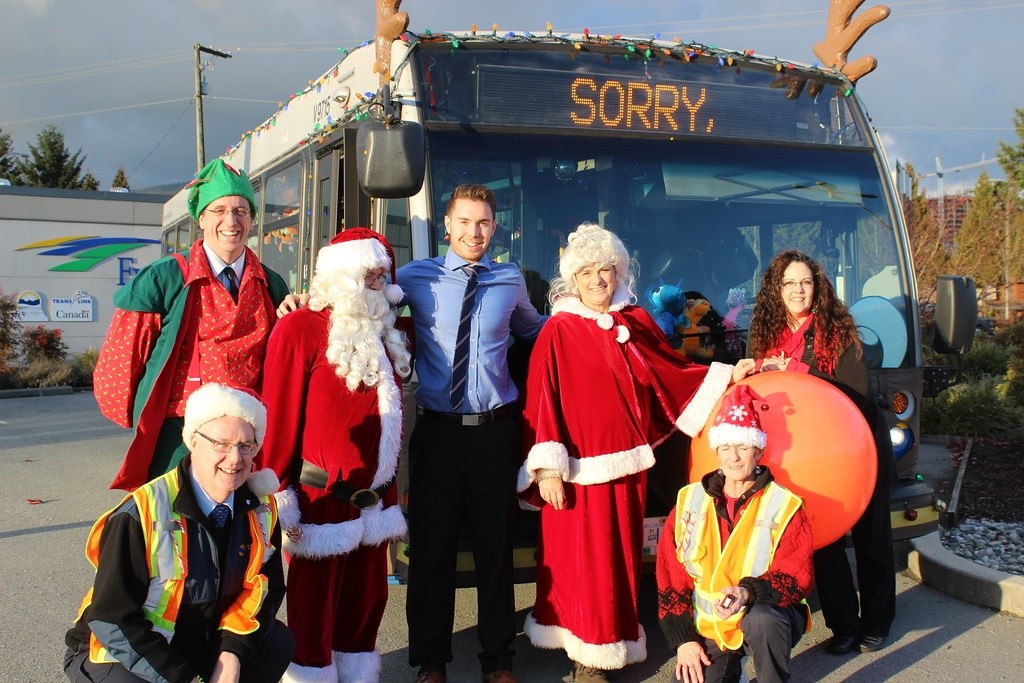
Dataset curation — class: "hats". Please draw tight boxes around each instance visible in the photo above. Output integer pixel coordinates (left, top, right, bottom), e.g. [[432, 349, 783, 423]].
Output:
[[183, 382, 268, 457], [709, 384, 769, 450], [184, 158, 257, 224], [316, 227, 403, 306]]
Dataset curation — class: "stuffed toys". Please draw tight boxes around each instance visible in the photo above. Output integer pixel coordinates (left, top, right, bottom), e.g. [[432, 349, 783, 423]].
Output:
[[645, 276, 755, 367]]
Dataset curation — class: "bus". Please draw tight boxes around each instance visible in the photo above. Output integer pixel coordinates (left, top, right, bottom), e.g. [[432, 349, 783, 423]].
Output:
[[162, 28, 977, 589]]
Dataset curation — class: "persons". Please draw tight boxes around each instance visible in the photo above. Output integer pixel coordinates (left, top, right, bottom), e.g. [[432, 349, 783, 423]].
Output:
[[745, 249, 895, 655], [247, 227, 416, 683], [93, 158, 290, 492], [64, 383, 292, 683], [515, 224, 756, 683], [276, 183, 550, 683], [656, 385, 816, 683]]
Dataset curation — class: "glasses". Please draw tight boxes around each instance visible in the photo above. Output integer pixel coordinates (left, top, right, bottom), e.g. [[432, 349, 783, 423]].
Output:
[[204, 207, 254, 220], [195, 430, 257, 454], [777, 278, 817, 290]]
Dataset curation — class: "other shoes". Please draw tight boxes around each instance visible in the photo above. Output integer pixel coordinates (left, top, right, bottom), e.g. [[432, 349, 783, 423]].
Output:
[[856, 633, 886, 653], [827, 631, 860, 654]]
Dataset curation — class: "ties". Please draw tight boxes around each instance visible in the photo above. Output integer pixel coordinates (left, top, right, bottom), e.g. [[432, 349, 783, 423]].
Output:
[[449, 263, 477, 410], [222, 268, 242, 302]]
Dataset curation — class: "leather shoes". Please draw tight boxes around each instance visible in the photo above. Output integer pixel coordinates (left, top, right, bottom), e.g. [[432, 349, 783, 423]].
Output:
[[417, 669, 444, 683], [482, 669, 515, 683]]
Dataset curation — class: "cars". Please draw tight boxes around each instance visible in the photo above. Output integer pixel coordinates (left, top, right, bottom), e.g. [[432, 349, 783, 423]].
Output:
[[976, 317, 999, 337]]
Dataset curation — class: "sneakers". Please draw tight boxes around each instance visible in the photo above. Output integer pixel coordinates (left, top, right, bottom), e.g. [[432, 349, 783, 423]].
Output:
[[572, 651, 609, 683]]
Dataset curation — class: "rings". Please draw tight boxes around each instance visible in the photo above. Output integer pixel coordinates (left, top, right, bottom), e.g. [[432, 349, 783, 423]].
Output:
[[731, 602, 741, 615], [689, 665, 695, 668]]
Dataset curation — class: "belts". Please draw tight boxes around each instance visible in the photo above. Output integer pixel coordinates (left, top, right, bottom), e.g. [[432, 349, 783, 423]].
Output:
[[417, 402, 518, 428], [291, 456, 397, 511]]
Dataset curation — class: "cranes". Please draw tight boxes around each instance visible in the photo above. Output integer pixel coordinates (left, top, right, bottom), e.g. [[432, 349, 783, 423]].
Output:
[[918, 153, 1014, 224]]
